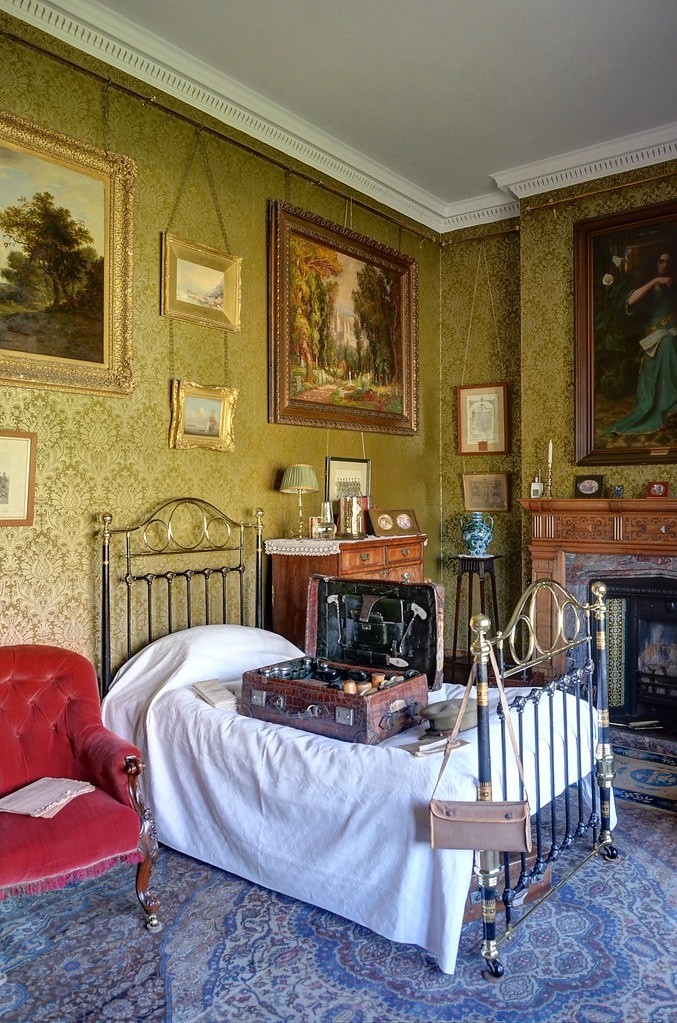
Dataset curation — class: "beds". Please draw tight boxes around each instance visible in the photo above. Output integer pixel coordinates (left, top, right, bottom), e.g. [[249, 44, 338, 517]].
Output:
[[98, 496, 617, 977]]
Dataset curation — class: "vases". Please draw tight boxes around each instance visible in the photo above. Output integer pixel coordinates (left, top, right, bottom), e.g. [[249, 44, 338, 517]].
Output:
[[461, 512, 494, 555]]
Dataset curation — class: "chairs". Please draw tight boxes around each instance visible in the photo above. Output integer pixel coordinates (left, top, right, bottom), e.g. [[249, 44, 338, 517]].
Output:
[[0, 644, 163, 934]]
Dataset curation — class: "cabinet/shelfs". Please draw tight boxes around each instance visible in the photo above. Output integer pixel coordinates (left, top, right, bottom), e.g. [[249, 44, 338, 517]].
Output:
[[268, 533, 429, 652]]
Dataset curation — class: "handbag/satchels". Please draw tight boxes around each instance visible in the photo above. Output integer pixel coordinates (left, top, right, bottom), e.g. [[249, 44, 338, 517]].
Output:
[[416, 697, 478, 740], [429, 800, 532, 852]]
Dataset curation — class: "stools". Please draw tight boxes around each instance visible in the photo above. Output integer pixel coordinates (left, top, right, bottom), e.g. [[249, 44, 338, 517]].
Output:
[[448, 556, 502, 684]]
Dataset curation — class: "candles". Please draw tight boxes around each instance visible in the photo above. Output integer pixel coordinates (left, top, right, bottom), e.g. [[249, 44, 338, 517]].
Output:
[[547, 440, 552, 468]]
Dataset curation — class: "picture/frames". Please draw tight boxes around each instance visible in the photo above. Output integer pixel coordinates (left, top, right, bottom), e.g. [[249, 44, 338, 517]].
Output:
[[160, 231, 243, 333], [168, 379, 240, 454], [0, 430, 37, 526], [574, 474, 604, 498], [572, 197, 677, 467], [647, 481, 668, 499], [325, 456, 371, 518], [462, 472, 510, 513], [267, 197, 421, 437], [0, 109, 134, 398], [368, 509, 421, 536]]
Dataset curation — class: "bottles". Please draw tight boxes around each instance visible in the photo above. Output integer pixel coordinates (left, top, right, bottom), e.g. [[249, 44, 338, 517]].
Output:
[[460, 511, 494, 557]]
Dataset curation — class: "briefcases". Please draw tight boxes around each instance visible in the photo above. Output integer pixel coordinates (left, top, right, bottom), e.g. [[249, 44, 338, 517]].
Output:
[[237, 571, 441, 746]]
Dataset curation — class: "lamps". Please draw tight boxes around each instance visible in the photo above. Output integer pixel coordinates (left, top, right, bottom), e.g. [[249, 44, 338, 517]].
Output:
[[279, 464, 319, 539]]
